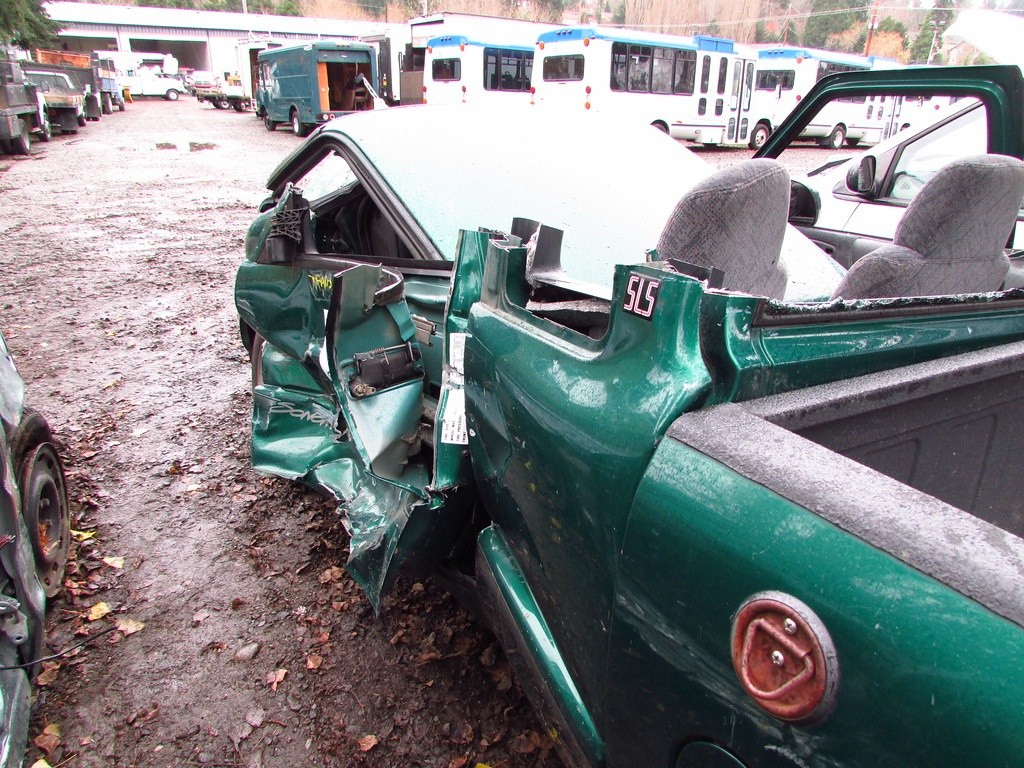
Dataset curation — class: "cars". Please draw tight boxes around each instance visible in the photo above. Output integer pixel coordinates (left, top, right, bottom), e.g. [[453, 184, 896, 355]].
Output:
[[0, 47, 186, 156]]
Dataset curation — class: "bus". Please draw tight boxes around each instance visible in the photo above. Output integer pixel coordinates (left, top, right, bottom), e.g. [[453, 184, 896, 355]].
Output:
[[754, 45, 971, 152], [421, 34, 535, 104], [528, 23, 759, 148]]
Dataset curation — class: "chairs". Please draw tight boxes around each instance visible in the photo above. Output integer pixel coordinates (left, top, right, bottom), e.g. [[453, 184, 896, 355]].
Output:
[[653, 158, 791, 304], [827, 153, 1024, 307]]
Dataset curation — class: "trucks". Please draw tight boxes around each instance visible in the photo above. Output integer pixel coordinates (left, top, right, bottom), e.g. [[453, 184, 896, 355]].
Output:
[[253, 47, 381, 137]]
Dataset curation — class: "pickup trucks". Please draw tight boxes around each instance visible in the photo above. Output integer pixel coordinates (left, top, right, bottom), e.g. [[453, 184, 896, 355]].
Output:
[[233, 63, 1023, 768], [193, 67, 250, 112]]
